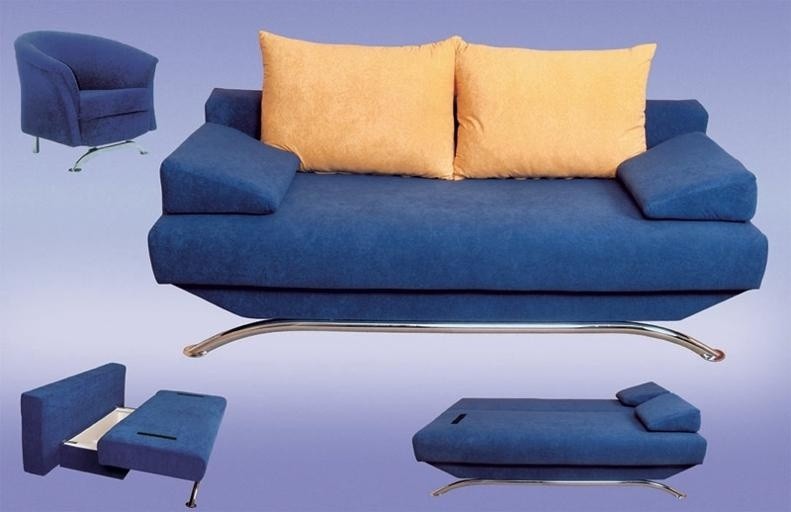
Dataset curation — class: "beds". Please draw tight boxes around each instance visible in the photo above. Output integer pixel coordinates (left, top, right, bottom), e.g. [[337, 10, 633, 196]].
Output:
[[413, 382, 705, 500]]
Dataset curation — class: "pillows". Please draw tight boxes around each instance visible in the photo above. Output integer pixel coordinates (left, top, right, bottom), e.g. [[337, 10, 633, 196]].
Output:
[[160, 122, 300, 216], [634, 395, 701, 433], [618, 133, 757, 223], [454, 42, 658, 181], [616, 382, 669, 406], [259, 31, 461, 182]]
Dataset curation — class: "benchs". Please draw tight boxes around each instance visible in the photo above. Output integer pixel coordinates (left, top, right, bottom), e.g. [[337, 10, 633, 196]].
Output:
[[20, 363, 226, 509]]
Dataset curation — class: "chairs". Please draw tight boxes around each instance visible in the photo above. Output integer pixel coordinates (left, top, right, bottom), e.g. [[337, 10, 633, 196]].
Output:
[[14, 31, 159, 172]]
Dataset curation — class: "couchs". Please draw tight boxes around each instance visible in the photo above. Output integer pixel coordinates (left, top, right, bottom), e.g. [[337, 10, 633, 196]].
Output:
[[147, 88, 767, 360]]
